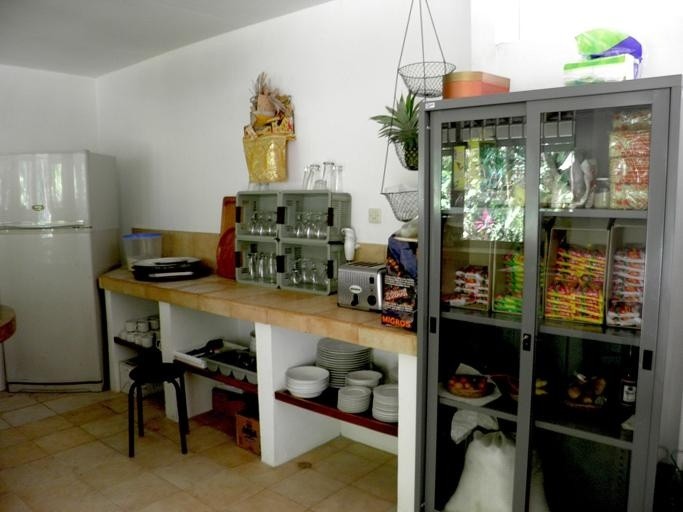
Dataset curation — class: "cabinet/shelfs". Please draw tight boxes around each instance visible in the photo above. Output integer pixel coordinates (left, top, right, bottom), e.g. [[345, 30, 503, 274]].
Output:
[[414, 77, 683, 509], [104, 289, 416, 510]]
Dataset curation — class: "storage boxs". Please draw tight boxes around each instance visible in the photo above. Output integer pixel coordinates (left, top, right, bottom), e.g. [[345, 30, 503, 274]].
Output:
[[234, 410, 259, 456]]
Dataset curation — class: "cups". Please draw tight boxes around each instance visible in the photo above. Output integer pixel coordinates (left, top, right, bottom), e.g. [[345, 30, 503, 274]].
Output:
[[246, 250, 277, 284], [302, 161, 342, 192]]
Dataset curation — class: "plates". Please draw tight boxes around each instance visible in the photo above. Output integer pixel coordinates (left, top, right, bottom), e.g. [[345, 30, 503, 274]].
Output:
[[284, 336, 398, 424]]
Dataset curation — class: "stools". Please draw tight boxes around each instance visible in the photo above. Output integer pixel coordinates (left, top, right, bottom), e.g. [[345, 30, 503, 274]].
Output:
[[124, 363, 190, 458]]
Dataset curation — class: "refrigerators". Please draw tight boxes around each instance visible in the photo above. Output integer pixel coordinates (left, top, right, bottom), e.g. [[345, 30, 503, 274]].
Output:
[[1, 150, 121, 395]]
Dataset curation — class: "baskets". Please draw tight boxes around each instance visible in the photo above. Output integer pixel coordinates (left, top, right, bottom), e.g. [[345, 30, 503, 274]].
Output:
[[397, 62, 456, 99], [393, 136, 418, 171], [381, 190, 420, 223]]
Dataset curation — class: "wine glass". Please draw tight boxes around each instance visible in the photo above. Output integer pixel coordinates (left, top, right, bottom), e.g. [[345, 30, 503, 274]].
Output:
[[288, 256, 329, 289], [242, 208, 328, 241]]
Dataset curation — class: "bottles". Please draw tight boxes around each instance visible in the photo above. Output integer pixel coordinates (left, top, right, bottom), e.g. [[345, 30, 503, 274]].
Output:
[[618, 344, 637, 410], [593, 177, 611, 208]]
